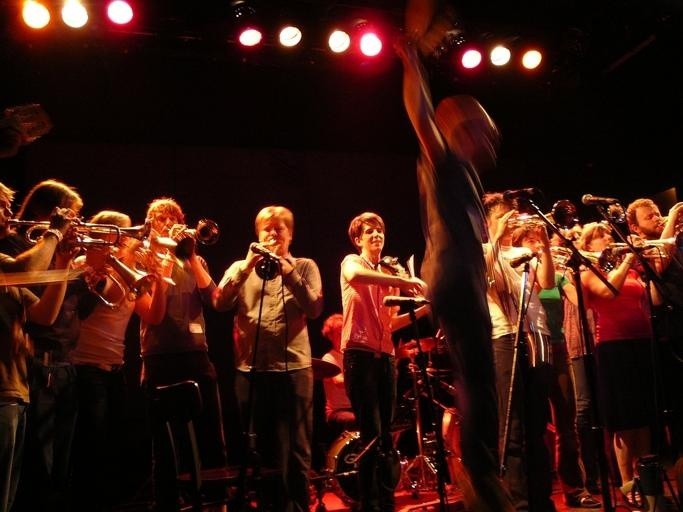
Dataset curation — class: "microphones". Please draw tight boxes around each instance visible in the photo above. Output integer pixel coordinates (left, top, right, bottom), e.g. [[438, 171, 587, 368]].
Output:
[[581, 194, 618, 206], [509, 253, 537, 267], [251, 243, 280, 261], [383, 296, 427, 307], [612, 245, 651, 256], [503, 188, 533, 200]]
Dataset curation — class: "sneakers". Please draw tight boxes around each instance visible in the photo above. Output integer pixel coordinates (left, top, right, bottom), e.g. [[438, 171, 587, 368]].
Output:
[[622, 491, 650, 510], [565, 490, 601, 508]]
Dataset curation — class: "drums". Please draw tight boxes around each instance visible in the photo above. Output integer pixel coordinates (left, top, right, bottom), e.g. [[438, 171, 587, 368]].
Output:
[[326, 431, 400, 507]]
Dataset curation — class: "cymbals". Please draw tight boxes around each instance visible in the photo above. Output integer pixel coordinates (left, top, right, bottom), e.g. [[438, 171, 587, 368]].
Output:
[[311, 358, 340, 378], [408, 368, 448, 373]]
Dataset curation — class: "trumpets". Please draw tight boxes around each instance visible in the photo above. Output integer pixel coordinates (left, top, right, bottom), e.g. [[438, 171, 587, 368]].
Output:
[[505, 200, 577, 228], [538, 231, 683, 275], [255, 239, 281, 281], [77, 233, 159, 308], [159, 219, 219, 267], [7, 218, 151, 248]]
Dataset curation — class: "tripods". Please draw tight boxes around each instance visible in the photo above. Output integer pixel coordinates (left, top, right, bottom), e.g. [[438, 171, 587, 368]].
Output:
[[392, 352, 458, 499], [196, 287, 285, 512]]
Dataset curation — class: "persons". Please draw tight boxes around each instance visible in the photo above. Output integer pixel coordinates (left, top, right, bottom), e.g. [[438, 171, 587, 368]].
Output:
[[0, 181, 223, 512], [483, 193, 682, 506], [322, 212, 427, 510], [211, 206, 322, 511], [392, 27, 508, 507]]
